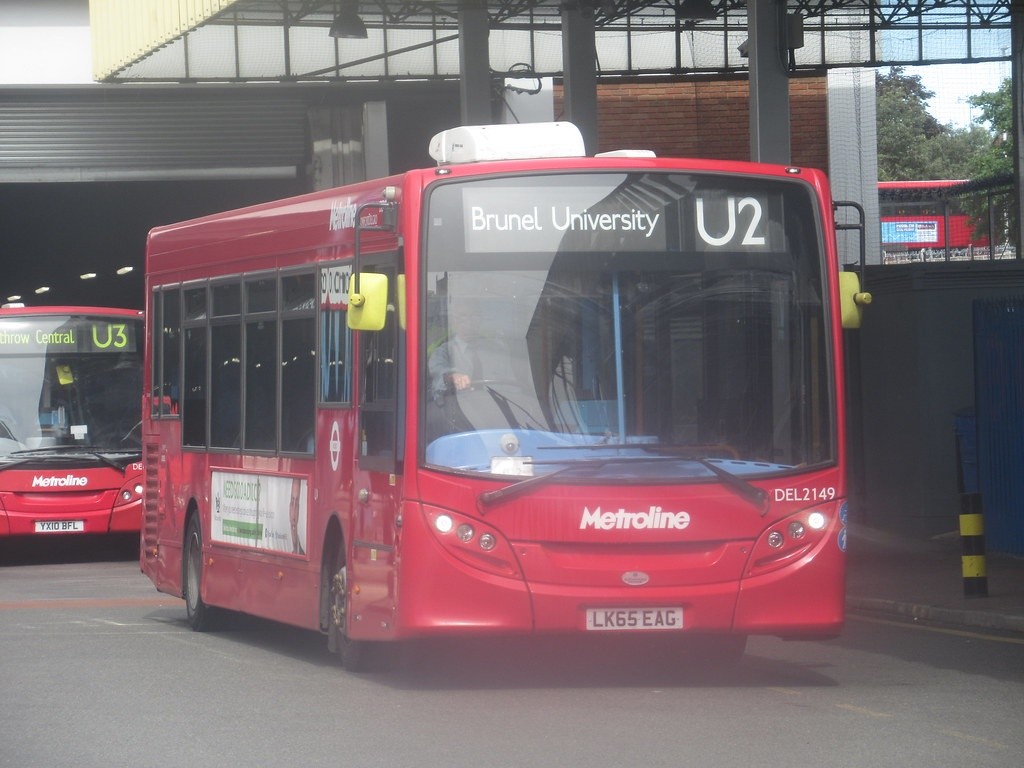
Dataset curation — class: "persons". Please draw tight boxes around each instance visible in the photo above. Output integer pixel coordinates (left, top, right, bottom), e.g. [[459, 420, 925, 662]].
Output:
[[429, 296, 514, 400]]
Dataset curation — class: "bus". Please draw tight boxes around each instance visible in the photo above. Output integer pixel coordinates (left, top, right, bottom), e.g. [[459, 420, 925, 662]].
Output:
[[139, 122, 871, 669], [0, 302, 146, 539], [879, 179, 1004, 253]]
